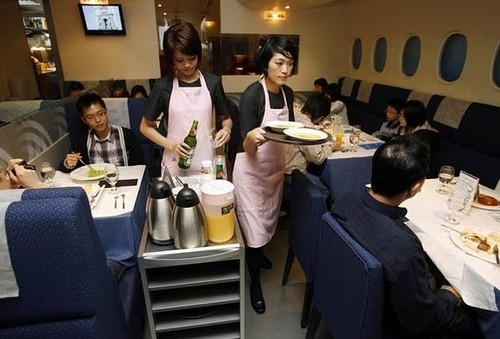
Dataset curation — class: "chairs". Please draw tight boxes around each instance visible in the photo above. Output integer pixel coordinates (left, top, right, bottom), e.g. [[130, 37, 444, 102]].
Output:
[[305, 214, 383, 339], [281, 169, 322, 329]]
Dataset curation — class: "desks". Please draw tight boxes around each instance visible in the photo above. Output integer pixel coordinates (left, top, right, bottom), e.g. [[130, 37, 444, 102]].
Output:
[[364, 177, 500, 313], [305, 124, 385, 193], [52, 165, 148, 267]]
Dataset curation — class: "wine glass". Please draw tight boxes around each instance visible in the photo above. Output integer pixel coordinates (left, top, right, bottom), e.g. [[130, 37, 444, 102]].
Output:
[[103, 163, 119, 194], [349, 124, 361, 152], [436, 165, 454, 194], [41, 161, 55, 188]]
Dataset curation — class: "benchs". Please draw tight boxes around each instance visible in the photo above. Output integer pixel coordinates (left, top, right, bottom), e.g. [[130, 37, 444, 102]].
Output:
[[113, 79, 159, 95], [0, 187, 146, 339], [332, 77, 500, 190], [65, 97, 146, 147], [0, 100, 59, 125]]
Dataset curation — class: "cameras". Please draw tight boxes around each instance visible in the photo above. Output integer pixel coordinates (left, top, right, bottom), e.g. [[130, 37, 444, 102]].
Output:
[[12, 160, 35, 176]]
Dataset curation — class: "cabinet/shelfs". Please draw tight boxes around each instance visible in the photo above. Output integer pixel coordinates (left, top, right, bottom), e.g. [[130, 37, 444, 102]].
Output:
[[137, 218, 246, 339]]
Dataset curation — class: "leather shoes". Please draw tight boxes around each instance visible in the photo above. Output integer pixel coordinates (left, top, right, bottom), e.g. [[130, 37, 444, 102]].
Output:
[[262, 255, 273, 269], [250, 288, 266, 314]]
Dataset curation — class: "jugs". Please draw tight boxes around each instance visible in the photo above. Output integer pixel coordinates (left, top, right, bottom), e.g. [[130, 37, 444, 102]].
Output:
[[171, 183, 207, 248], [145, 180, 175, 245], [200, 179, 236, 243]]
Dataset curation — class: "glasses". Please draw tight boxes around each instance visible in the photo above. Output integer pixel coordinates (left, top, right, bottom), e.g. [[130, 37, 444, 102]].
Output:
[[82, 110, 106, 121]]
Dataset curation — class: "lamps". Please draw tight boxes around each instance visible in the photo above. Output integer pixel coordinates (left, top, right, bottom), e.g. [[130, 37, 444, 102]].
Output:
[[200, 17, 216, 50]]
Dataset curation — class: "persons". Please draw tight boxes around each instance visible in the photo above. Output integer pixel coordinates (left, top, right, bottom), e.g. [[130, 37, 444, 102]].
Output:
[[0, 159, 127, 285], [332, 134, 485, 339], [232, 35, 296, 315], [59, 93, 162, 178], [285, 93, 330, 200], [379, 98, 439, 141], [314, 78, 349, 125], [63, 79, 146, 98], [140, 22, 234, 179]]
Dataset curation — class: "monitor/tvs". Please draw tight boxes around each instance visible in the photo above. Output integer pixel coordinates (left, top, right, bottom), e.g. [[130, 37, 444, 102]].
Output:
[[80, 3, 127, 36]]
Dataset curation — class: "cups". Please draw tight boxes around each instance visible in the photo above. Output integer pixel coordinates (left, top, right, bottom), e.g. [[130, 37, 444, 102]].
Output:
[[336, 132, 344, 141]]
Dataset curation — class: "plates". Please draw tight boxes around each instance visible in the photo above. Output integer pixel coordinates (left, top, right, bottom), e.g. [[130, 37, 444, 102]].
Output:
[[282, 128, 329, 142], [70, 163, 115, 180], [265, 119, 305, 132], [451, 221, 500, 264], [472, 192, 500, 210]]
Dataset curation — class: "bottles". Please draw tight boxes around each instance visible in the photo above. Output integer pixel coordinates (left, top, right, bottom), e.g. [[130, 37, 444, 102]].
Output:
[[178, 120, 199, 169], [202, 155, 228, 182]]
